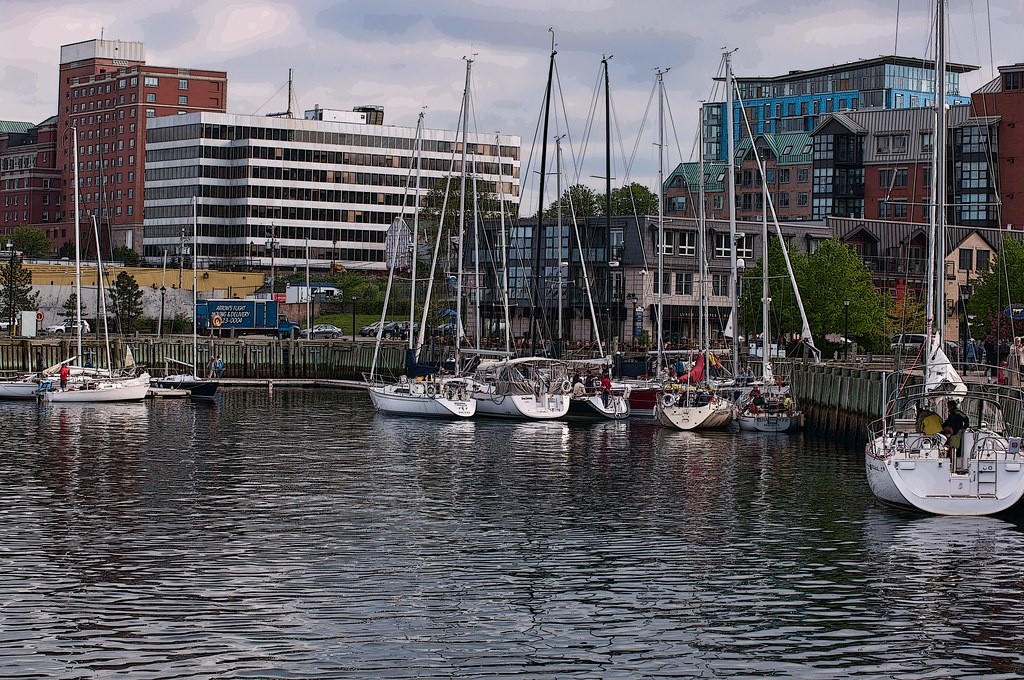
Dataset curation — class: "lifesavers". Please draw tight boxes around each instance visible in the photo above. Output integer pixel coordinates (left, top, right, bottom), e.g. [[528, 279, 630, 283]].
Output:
[[36, 312, 44, 321], [788, 402, 793, 414], [662, 394, 676, 407], [212, 315, 222, 327], [426, 385, 436, 398], [561, 380, 572, 392]]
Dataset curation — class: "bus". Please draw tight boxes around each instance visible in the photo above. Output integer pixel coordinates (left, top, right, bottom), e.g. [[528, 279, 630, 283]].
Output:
[[0, 251, 23, 259]]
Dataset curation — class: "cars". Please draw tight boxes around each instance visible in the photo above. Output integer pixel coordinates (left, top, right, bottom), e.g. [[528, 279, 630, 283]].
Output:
[[0, 316, 18, 331], [358, 320, 432, 339], [45, 318, 91, 335], [60, 257, 69, 260], [300, 323, 344, 339]]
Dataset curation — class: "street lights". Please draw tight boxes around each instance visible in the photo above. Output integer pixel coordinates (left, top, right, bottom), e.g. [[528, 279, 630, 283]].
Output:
[[737, 258, 746, 348], [626, 293, 639, 352], [607, 260, 619, 352], [550, 262, 569, 338], [843, 299, 851, 360], [265, 223, 278, 301]]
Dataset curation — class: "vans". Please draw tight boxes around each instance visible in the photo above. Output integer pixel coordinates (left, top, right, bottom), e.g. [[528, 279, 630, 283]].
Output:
[[889, 332, 927, 353]]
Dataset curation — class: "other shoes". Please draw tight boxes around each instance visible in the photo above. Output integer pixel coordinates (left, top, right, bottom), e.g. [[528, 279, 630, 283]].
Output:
[[969, 367, 974, 372]]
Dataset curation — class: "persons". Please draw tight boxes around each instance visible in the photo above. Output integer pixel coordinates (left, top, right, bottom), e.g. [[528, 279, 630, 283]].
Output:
[[783, 392, 791, 410], [601, 373, 611, 409], [915, 407, 944, 436], [36, 351, 44, 379], [749, 383, 761, 403], [942, 400, 969, 457], [85, 358, 91, 366], [572, 370, 582, 385], [736, 366, 754, 387], [585, 370, 598, 397], [207, 356, 217, 380], [60, 363, 70, 392], [599, 364, 610, 378], [964, 335, 1024, 384], [675, 357, 683, 378], [215, 355, 223, 379], [670, 385, 715, 407], [573, 379, 586, 397]]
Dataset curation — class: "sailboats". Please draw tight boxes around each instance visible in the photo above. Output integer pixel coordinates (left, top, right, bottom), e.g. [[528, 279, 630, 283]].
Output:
[[438, 43, 573, 420], [616, 44, 806, 434], [506, 26, 632, 421], [148, 196, 222, 400], [864, 1, 1024, 517], [41, 214, 151, 405], [540, 133, 613, 375], [0, 127, 145, 401], [364, 117, 478, 421]]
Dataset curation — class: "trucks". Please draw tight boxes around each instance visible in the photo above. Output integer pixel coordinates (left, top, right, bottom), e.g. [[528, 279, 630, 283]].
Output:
[[191, 297, 300, 340]]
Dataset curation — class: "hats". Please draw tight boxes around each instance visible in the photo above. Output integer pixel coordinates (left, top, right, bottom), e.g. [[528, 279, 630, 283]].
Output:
[[209, 356, 214, 359], [969, 338, 974, 341]]
